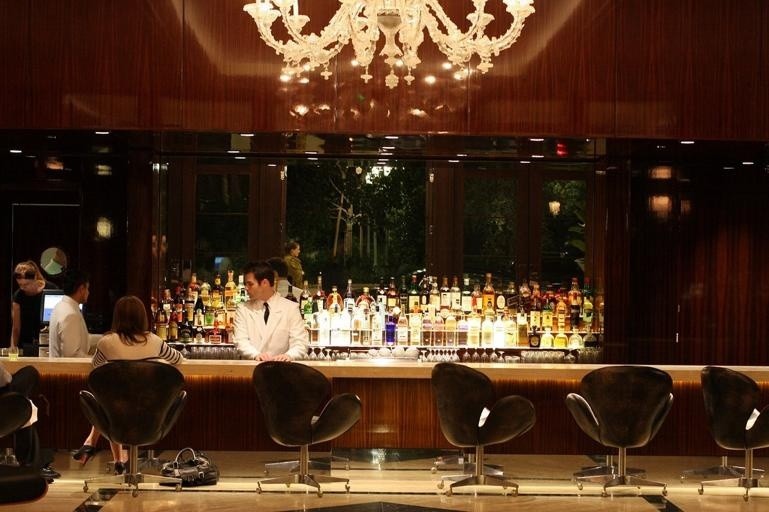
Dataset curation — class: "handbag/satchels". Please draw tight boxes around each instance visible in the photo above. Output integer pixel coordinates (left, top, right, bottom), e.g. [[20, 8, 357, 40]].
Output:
[[159, 446, 220, 487]]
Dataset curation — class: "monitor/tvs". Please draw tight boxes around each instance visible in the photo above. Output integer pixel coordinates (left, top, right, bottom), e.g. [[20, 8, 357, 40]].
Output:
[[39, 289, 83, 326]]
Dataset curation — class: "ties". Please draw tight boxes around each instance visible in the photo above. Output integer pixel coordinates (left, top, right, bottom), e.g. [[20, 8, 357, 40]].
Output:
[[264, 303, 269, 324]]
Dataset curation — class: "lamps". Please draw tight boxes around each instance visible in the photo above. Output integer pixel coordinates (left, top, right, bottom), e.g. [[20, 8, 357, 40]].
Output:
[[356, 163, 363, 177], [428, 169, 435, 185], [281, 169, 287, 180], [233, 0, 541, 94]]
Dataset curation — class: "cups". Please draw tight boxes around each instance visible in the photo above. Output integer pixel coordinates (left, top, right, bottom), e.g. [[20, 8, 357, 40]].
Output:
[[505, 347, 602, 363], [8, 347, 19, 359], [190, 346, 242, 360]]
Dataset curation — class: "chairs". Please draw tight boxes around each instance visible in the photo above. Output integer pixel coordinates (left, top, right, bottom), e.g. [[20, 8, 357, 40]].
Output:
[[1, 466, 49, 508], [677, 361, 769, 506], [78, 351, 195, 502], [420, 357, 537, 500], [1, 389, 57, 484], [248, 358, 366, 498], [557, 359, 678, 499]]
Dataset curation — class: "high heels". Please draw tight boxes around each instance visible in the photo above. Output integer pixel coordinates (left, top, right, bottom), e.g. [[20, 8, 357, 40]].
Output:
[[71, 445, 97, 466], [114, 462, 126, 475]]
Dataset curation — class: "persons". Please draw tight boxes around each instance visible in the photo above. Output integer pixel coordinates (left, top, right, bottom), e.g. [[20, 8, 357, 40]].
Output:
[[8, 260, 62, 353], [48, 267, 93, 360], [282, 237, 305, 289], [68, 294, 185, 476], [264, 258, 307, 303], [231, 264, 310, 363]]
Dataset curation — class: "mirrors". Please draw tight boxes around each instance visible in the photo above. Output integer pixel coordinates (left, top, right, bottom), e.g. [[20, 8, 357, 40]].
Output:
[[40, 246, 69, 275]]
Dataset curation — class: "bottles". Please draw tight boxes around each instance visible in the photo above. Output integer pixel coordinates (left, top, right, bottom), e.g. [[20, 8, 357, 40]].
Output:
[[154, 265, 596, 353]]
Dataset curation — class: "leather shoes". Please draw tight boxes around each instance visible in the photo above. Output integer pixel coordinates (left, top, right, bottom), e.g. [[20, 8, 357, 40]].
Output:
[[41, 467, 62, 480]]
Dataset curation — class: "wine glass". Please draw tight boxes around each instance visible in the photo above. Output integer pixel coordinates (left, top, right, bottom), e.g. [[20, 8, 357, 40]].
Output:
[[424, 345, 504, 365], [180, 343, 189, 358], [306, 344, 339, 364]]
